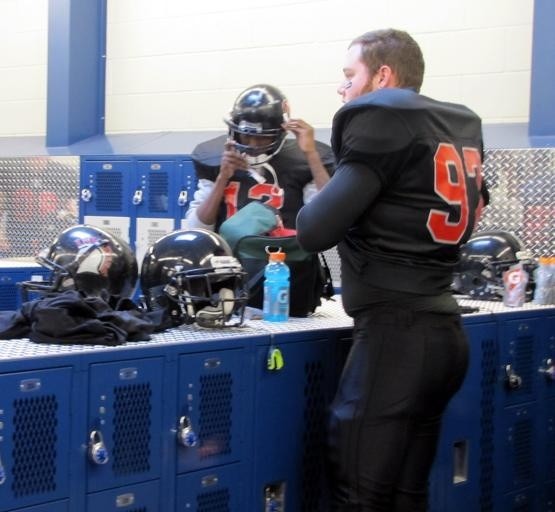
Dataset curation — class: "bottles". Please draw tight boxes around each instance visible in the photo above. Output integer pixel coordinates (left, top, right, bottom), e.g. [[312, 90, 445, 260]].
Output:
[[263, 252, 290, 321]]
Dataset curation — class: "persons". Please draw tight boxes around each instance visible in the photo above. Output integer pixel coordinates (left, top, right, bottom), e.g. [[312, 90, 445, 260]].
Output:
[[179, 83, 337, 234], [295, 27, 491, 512]]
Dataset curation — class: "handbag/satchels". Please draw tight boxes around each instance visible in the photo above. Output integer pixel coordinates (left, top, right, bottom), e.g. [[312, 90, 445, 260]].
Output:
[[235, 234, 327, 317]]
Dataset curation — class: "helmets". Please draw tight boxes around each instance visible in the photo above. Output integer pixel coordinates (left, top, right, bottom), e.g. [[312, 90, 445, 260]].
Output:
[[224, 84, 292, 167], [22, 225, 138, 312], [452, 232, 536, 302], [141, 228, 249, 328]]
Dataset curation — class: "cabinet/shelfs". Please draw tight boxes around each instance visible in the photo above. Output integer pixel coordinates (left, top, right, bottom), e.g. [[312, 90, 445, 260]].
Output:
[[78, 154, 201, 303], [1, 294, 554, 512], [2, 271, 58, 312]]
